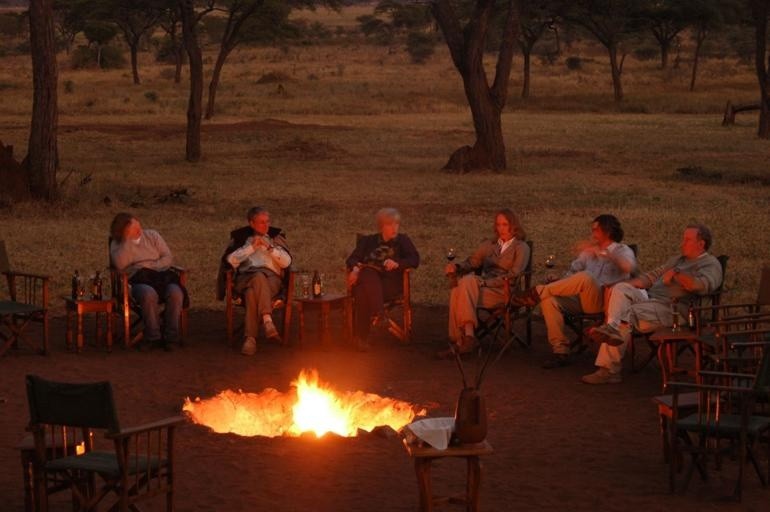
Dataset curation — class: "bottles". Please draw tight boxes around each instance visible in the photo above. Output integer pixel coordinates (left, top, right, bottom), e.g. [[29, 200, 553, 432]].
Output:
[[72, 269, 103, 301], [670, 299, 694, 333], [295, 269, 327, 299]]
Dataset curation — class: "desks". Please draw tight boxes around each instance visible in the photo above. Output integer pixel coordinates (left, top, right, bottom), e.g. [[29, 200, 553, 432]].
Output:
[[403, 435, 493, 512], [17, 434, 102, 509]]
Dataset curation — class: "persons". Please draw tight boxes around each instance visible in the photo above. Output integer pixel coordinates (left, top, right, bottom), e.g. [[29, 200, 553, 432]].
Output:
[[109, 212, 184, 349], [345, 207, 420, 348], [581, 222, 724, 384], [510, 213, 638, 368], [435, 208, 531, 361], [226, 206, 293, 356]]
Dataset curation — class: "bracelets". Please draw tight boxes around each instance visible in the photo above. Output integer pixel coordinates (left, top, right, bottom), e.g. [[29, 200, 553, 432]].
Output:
[[267, 244, 274, 250]]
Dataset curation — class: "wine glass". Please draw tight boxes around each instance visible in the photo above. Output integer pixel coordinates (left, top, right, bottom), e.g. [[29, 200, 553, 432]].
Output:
[[447, 248, 456, 274], [543, 254, 556, 283]]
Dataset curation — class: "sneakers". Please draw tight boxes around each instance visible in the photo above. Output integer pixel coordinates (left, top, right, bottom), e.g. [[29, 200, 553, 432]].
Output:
[[588, 323, 624, 346], [542, 353, 571, 368], [455, 334, 480, 354], [263, 320, 279, 338], [582, 367, 623, 385], [369, 314, 392, 329], [137, 335, 161, 353], [355, 335, 368, 352], [240, 335, 257, 356], [434, 345, 456, 360], [510, 286, 539, 307], [159, 339, 176, 352]]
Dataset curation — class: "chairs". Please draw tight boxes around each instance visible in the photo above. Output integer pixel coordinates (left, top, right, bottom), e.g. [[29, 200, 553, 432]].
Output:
[[0, 232, 190, 357], [26, 375, 184, 510], [222, 231, 417, 351]]
